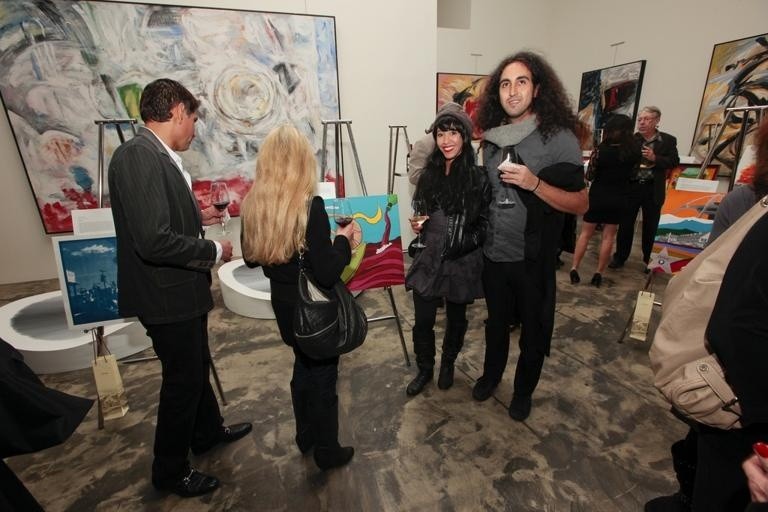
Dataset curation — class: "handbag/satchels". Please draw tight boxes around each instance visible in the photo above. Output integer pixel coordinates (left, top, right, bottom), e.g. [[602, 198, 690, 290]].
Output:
[[649, 194, 768, 431], [629, 290, 656, 342], [292, 267, 368, 361], [93, 355, 128, 422]]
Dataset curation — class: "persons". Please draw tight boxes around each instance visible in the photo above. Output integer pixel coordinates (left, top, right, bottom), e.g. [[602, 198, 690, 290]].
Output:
[[704, 112, 768, 241], [401, 102, 490, 394], [107, 79, 252, 496], [650, 193, 768, 511], [608, 106, 677, 268], [473, 51, 589, 421], [239, 126, 356, 468], [741, 440, 768, 509], [407, 134, 478, 187], [570, 114, 634, 288]]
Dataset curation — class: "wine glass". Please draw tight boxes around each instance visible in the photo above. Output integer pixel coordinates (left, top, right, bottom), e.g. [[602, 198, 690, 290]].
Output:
[[641, 144, 649, 163], [410, 199, 427, 248], [333, 198, 353, 228], [210, 182, 231, 236], [497, 146, 517, 205]]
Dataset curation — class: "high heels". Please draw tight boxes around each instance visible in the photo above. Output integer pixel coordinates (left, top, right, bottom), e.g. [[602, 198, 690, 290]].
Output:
[[570, 269, 579, 285], [591, 273, 601, 288]]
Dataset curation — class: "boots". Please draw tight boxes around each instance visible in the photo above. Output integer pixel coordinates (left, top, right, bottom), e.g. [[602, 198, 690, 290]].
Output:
[[438, 320, 469, 390], [312, 395, 354, 470], [406, 329, 436, 395], [290, 381, 316, 455]]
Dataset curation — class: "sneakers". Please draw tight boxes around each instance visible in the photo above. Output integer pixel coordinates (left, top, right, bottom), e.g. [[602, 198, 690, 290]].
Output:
[[509, 392, 531, 421]]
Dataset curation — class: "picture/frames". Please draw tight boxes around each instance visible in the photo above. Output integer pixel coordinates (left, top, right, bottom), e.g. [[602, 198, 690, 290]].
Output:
[[689, 31, 768, 180], [0, 0, 344, 237], [576, 59, 647, 160], [666, 162, 721, 189], [435, 71, 495, 143]]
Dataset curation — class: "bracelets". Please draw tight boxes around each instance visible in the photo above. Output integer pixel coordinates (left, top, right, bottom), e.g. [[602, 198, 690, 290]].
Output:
[[530, 177, 540, 192]]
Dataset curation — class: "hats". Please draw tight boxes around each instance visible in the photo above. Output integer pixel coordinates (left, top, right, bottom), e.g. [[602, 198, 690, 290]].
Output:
[[433, 101, 473, 137]]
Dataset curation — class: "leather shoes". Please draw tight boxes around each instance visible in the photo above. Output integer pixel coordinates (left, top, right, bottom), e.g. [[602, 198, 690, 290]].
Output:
[[607, 261, 624, 269], [152, 467, 219, 497], [190, 422, 252, 456], [473, 377, 501, 401]]
